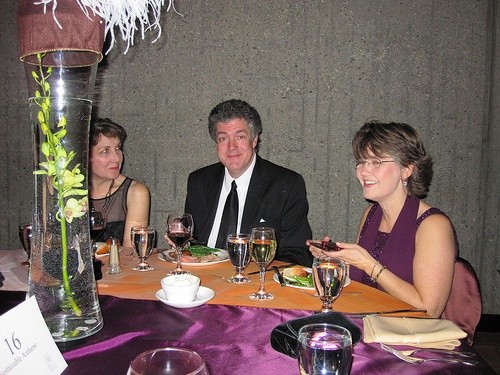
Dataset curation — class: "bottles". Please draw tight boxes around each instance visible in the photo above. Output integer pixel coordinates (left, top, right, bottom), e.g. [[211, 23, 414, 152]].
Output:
[[107, 238, 122, 275]]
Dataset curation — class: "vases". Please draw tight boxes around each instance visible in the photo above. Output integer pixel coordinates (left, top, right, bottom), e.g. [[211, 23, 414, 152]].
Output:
[[23, 51, 104, 342]]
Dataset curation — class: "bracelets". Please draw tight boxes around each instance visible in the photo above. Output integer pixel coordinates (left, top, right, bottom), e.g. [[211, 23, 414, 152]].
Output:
[[370, 260, 379, 278], [374, 266, 387, 282]]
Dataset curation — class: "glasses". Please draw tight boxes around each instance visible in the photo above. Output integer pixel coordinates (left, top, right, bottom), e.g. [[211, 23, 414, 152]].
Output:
[[355, 157, 394, 168]]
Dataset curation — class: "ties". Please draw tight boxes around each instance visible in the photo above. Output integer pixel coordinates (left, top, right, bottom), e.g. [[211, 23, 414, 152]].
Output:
[[215, 180, 239, 252]]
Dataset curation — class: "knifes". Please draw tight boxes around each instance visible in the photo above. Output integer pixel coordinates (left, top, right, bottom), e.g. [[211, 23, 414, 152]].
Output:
[[157, 247, 175, 264], [271, 265, 286, 287]]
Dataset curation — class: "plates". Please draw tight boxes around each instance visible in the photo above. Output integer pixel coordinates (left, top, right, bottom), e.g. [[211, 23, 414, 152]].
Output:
[[273, 266, 351, 290], [158, 247, 231, 264], [155, 285, 216, 309], [94, 241, 122, 258]]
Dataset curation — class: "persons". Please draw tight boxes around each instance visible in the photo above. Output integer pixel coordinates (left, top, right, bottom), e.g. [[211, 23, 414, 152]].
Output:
[[89, 118, 150, 247], [306, 121, 459, 319], [164, 99, 314, 268]]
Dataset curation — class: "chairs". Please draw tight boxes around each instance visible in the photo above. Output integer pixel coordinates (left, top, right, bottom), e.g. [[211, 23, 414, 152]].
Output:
[[441, 259, 482, 347]]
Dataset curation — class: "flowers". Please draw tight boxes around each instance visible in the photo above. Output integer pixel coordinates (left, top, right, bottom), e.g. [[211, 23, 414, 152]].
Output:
[[32, 53, 89, 316]]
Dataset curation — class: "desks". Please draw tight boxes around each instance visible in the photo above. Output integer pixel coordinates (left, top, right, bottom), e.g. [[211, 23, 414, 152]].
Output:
[[0, 246, 498, 375]]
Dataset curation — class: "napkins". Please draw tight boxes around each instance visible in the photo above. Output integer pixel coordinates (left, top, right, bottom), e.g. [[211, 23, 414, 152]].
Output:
[[363, 315, 467, 350]]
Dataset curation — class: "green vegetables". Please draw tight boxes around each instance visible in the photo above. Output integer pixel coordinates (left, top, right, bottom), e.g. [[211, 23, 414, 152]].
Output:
[[285, 273, 333, 287], [182, 244, 221, 256]]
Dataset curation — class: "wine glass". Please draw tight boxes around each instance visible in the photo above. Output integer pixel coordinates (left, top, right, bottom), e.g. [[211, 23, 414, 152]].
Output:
[[312, 256, 347, 313], [227, 233, 254, 283], [18, 224, 33, 266], [166, 212, 195, 274], [89, 208, 105, 251], [250, 227, 275, 300], [129, 226, 156, 272]]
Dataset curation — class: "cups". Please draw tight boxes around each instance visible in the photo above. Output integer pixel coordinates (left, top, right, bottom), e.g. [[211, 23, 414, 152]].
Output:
[[125, 347, 210, 375], [160, 274, 201, 304], [296, 322, 353, 375]]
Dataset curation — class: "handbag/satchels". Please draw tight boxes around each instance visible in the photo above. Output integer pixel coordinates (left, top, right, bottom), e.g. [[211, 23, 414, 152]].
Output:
[[270, 311, 362, 359]]
